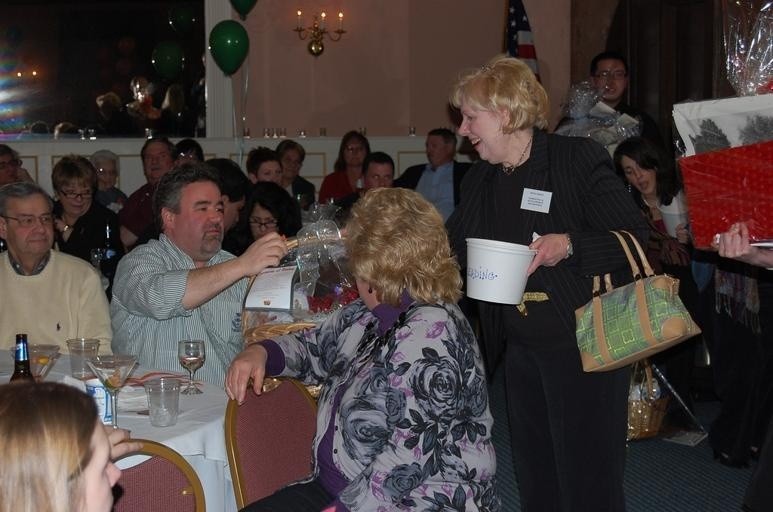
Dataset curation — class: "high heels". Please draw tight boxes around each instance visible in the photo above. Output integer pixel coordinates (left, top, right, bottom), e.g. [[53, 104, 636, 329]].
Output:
[[709, 434, 752, 468]]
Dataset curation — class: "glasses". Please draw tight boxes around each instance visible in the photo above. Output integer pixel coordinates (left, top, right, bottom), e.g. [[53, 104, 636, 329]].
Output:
[[1, 213, 57, 229], [179, 152, 197, 161], [59, 186, 94, 200], [97, 167, 117, 175], [245, 218, 279, 230], [594, 69, 626, 82], [1, 160, 24, 170]]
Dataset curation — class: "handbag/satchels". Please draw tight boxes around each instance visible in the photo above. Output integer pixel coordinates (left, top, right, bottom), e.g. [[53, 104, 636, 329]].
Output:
[[574, 273, 702, 374]]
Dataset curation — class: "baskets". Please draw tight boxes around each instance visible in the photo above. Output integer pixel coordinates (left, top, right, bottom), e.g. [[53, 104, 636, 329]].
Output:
[[240, 235, 346, 395], [626, 358, 673, 442]]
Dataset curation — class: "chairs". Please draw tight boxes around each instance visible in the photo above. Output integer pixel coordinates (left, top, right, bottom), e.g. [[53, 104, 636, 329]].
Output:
[[111, 439, 205, 512], [225, 376, 318, 510]]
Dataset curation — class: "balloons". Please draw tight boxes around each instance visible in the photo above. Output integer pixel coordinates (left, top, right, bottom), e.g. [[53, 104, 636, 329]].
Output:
[[151, 40, 185, 82], [229, 0, 256, 22], [209, 20, 249, 76], [168, 2, 201, 40]]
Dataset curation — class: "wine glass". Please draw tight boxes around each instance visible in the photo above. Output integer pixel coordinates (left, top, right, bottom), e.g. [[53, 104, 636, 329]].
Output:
[[85, 353, 139, 428], [176, 339, 207, 396]]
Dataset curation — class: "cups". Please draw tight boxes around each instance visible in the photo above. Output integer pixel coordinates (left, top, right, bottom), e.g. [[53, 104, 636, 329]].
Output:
[[144, 126, 152, 138], [9, 343, 61, 380], [262, 126, 285, 139], [65, 339, 105, 378], [144, 377, 181, 427], [462, 236, 538, 306], [654, 193, 690, 240], [78, 128, 96, 140]]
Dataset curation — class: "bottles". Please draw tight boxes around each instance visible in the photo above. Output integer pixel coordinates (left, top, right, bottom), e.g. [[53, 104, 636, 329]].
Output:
[[8, 333, 34, 383], [360, 127, 366, 137], [319, 128, 326, 138], [407, 126, 416, 136], [298, 131, 305, 138], [242, 127, 249, 139], [102, 223, 119, 261]]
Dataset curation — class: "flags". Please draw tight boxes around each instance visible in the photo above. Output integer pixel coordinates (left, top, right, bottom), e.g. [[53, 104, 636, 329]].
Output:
[[504, 0, 540, 83]]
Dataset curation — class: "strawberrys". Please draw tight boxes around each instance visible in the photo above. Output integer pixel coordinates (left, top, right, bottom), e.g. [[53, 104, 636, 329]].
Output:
[[306, 284, 360, 312]]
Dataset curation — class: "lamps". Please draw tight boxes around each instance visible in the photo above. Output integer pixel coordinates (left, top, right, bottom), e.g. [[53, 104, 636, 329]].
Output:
[[292, 9, 347, 56]]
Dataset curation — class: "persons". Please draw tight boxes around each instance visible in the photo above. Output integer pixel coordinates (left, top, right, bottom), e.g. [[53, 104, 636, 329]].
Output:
[[0, 51, 773, 512]]
